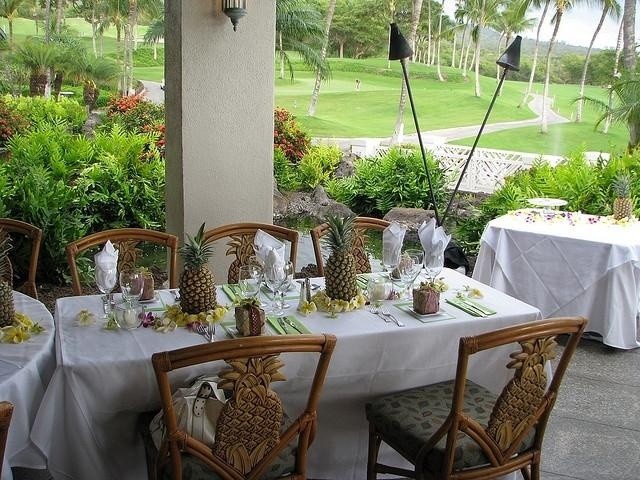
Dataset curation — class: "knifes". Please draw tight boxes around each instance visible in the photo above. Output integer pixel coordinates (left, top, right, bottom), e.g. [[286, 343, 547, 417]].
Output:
[[228, 285, 242, 299], [460, 300, 491, 318], [278, 317, 290, 335], [102, 295, 114, 314], [283, 316, 304, 335]]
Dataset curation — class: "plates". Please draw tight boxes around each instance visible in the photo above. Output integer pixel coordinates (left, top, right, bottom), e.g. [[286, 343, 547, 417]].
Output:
[[408, 307, 442, 318], [226, 327, 273, 339], [138, 298, 156, 304], [526, 198, 567, 206]]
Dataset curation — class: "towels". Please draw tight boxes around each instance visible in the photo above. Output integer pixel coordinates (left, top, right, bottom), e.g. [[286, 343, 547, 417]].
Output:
[[382, 220, 406, 262], [250, 227, 288, 267], [91, 238, 121, 269], [417, 218, 452, 269]]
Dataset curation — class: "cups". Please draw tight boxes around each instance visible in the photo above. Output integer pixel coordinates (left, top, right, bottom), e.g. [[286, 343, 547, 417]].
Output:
[[141, 272, 155, 300], [113, 303, 145, 330], [119, 268, 144, 304], [238, 266, 262, 298], [367, 278, 394, 300]]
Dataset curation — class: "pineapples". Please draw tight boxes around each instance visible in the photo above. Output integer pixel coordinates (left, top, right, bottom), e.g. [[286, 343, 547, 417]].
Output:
[[350, 248, 372, 274], [213, 391, 283, 461], [486, 372, 547, 439], [179, 266, 217, 314], [0, 256, 14, 286], [613, 198, 632, 222], [111, 263, 133, 292], [228, 259, 263, 284], [0, 283, 15, 328], [325, 252, 357, 302]]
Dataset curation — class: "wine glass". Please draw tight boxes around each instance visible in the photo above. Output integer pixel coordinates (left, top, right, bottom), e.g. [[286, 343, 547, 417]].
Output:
[[94, 253, 116, 321], [380, 246, 443, 301], [249, 256, 293, 315]]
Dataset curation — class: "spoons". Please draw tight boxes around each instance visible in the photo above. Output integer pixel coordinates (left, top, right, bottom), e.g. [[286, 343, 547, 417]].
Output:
[[296, 279, 321, 290]]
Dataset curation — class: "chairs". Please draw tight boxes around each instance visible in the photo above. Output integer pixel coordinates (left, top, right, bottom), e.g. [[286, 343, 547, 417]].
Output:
[[0, 217, 45, 300], [0, 399, 14, 479], [364, 312, 590, 479], [66, 226, 179, 297], [138, 331, 340, 480], [190, 220, 300, 285], [310, 213, 397, 277]]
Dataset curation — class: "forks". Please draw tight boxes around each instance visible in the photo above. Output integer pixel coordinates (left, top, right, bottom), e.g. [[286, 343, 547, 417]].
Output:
[[196, 323, 218, 343], [368, 304, 391, 323], [380, 306, 404, 327], [170, 290, 181, 302]]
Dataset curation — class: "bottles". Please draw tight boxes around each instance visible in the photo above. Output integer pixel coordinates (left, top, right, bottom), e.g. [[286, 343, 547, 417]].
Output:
[[295, 277, 311, 311]]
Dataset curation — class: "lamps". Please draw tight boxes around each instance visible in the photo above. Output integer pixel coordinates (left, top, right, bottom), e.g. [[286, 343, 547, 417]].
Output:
[[221, 0, 248, 34]]
